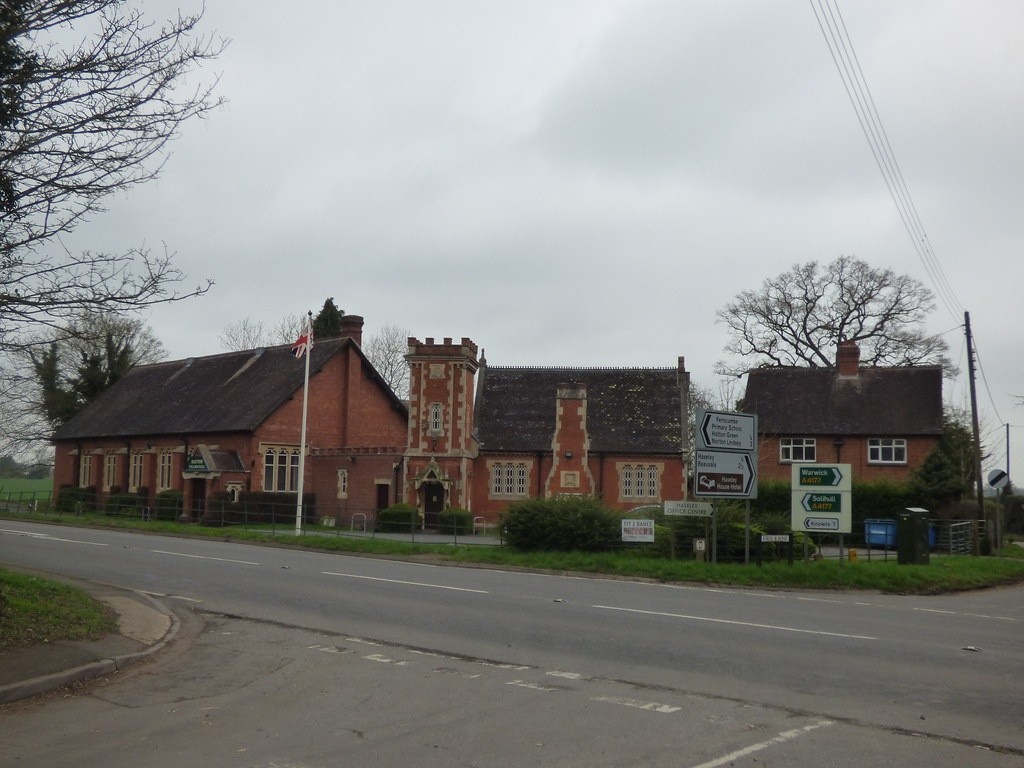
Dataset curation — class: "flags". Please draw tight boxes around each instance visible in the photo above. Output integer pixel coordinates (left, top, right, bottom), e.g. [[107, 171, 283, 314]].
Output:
[[290, 321, 314, 359]]
